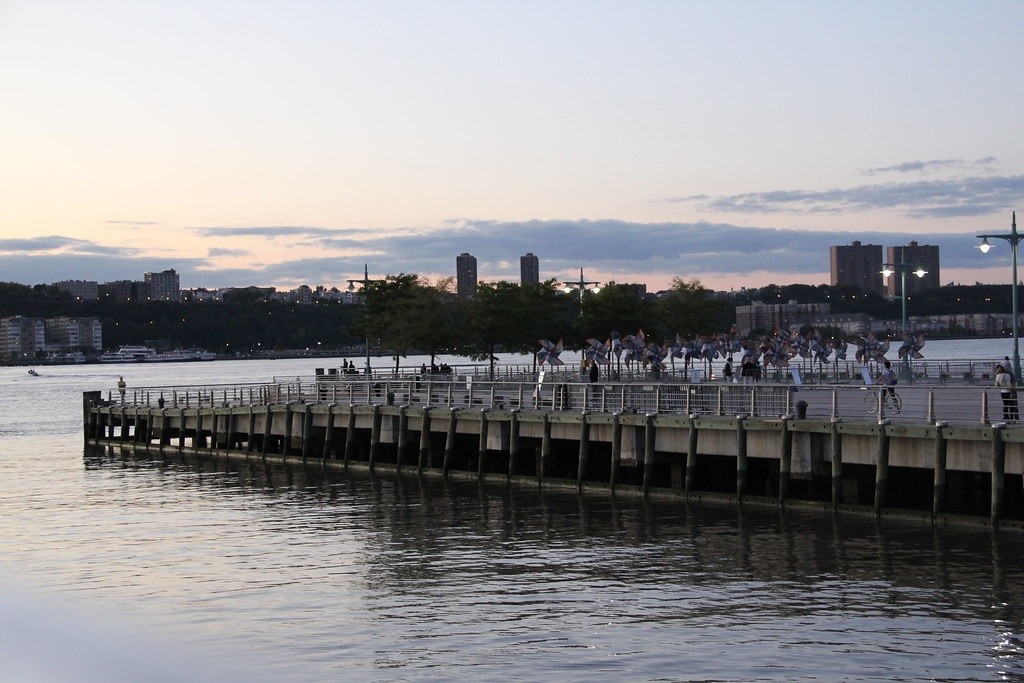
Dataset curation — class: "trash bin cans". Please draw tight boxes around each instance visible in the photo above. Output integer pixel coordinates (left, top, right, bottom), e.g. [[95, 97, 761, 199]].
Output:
[[796, 401, 809, 419], [158, 398, 165, 408]]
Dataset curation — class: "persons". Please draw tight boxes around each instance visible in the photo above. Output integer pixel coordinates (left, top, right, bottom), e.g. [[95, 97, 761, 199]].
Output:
[[877, 362, 900, 413], [349, 361, 355, 372], [118, 377, 126, 394], [421, 364, 426, 374], [742, 357, 761, 390], [725, 358, 735, 382], [589, 360, 598, 382], [343, 359, 348, 371], [995, 356, 1020, 420], [433, 363, 451, 373]]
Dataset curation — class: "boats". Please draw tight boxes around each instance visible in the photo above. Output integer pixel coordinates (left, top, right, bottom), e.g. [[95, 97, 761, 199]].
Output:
[[44, 350, 87, 365], [96, 344, 218, 364]]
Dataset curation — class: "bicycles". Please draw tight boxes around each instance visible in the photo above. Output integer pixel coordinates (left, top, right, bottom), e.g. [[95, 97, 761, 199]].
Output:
[[863, 383, 902, 415]]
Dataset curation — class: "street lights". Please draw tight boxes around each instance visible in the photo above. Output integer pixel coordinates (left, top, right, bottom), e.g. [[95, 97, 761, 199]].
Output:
[[874, 246, 930, 370], [346, 262, 386, 378], [564, 267, 601, 361], [972, 210, 1024, 382]]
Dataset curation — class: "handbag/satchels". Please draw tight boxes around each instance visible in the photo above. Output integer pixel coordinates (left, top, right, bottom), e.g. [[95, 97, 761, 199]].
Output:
[[733, 377, 737, 383]]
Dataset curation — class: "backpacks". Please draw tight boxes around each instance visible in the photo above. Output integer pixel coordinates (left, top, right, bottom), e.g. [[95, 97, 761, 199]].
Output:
[[887, 369, 897, 385]]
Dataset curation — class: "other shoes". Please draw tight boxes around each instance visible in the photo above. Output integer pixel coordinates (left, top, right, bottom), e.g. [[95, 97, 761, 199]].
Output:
[[895, 410, 901, 414]]
